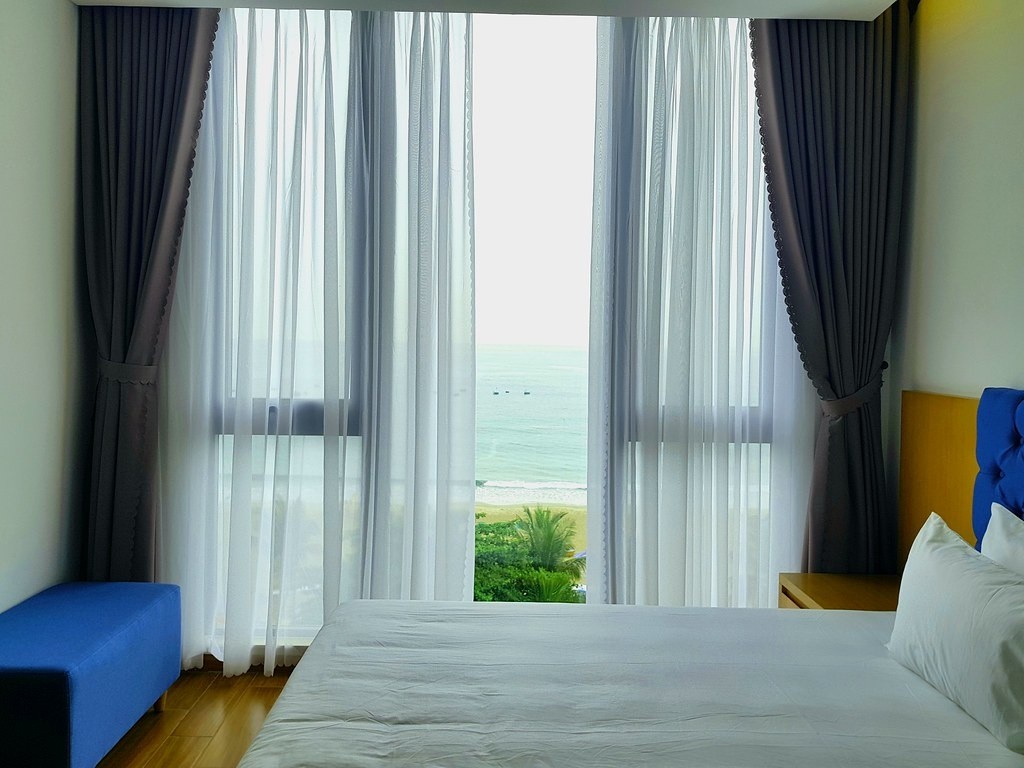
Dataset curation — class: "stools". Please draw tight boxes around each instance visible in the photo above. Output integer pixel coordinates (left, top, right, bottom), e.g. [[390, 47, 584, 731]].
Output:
[[0, 579, 182, 768]]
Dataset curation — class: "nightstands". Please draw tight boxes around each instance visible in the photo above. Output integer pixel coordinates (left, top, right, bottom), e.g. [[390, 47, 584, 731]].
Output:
[[778, 570, 901, 612]]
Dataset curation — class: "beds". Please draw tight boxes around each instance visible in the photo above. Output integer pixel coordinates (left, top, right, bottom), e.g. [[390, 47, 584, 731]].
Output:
[[235, 598, 1024, 768]]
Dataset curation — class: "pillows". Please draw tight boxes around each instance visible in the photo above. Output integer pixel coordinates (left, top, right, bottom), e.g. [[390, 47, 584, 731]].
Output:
[[980, 502, 1024, 575], [884, 508, 1024, 756]]
